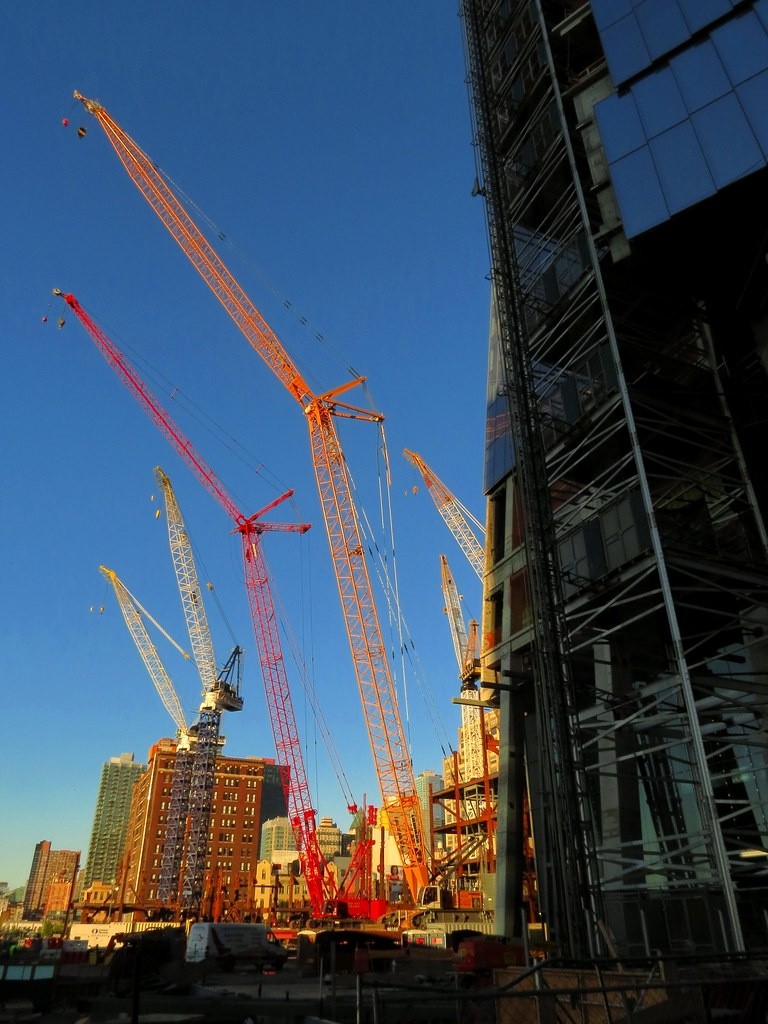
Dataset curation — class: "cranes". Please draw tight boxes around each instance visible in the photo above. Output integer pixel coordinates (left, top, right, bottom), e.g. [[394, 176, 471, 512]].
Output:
[[150, 466, 246, 923], [402, 447, 485, 888], [89, 564, 199, 923]]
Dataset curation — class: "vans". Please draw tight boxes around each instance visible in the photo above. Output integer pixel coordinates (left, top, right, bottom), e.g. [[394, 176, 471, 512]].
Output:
[[313, 927, 400, 973], [183, 923, 289, 974]]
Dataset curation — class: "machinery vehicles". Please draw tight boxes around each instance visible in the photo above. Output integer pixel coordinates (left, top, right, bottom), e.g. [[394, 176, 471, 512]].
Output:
[[41, 285, 402, 954], [61, 88, 494, 934]]
